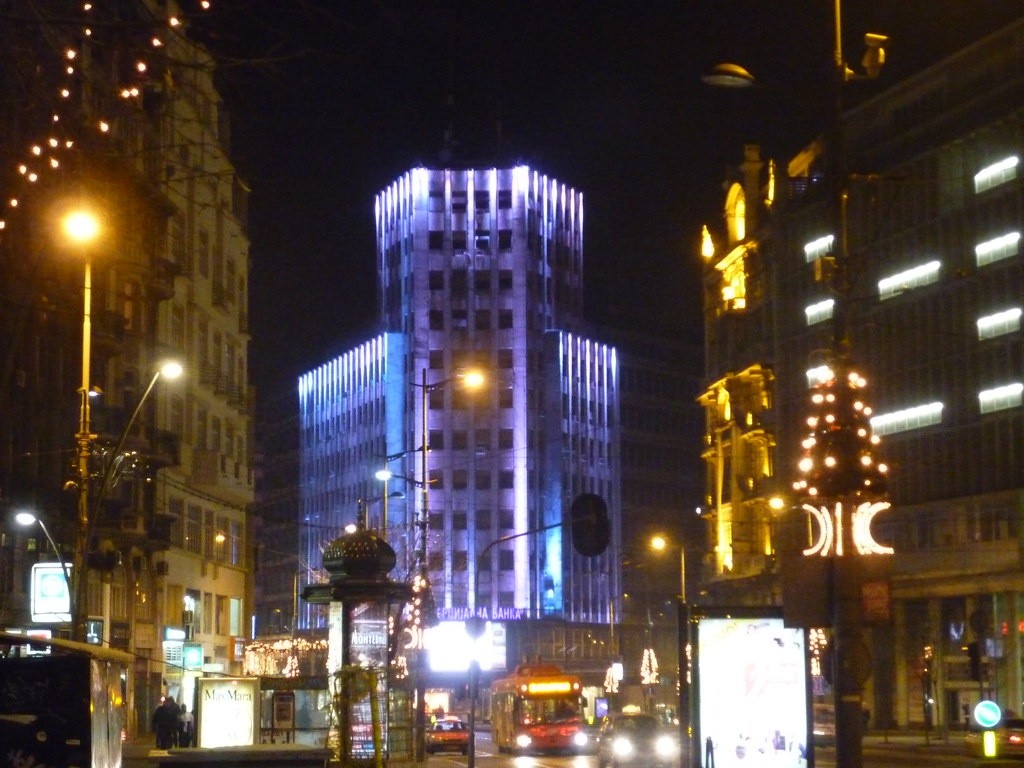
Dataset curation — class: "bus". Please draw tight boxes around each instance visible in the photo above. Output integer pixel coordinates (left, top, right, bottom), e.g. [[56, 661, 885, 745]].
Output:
[[489, 664, 588, 754], [0, 632, 138, 759]]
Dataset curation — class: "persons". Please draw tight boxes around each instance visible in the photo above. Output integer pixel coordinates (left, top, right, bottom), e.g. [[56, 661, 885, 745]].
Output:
[[0, 660, 87, 768], [150, 697, 194, 749]]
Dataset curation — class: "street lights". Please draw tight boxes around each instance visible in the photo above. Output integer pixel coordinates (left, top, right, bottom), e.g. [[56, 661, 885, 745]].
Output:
[[210, 530, 331, 585], [414, 368, 486, 569], [59, 203, 103, 640], [784, 335, 877, 768], [650, 532, 693, 767], [373, 469, 431, 763], [81, 359, 186, 626], [13, 511, 77, 634], [695, 54, 872, 357]]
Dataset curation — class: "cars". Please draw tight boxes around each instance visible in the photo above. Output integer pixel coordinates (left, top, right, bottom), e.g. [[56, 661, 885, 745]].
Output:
[[423, 721, 475, 756], [593, 705, 688, 768]]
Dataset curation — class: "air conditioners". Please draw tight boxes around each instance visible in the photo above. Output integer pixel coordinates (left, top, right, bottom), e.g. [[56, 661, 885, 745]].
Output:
[[106, 548, 195, 642]]
